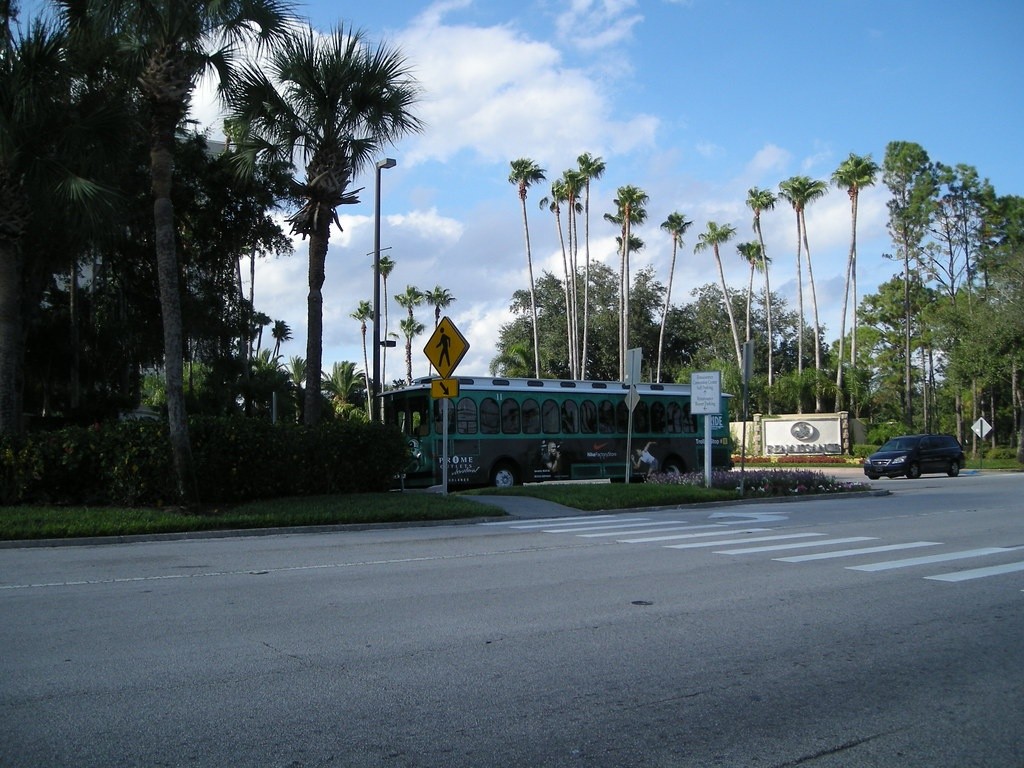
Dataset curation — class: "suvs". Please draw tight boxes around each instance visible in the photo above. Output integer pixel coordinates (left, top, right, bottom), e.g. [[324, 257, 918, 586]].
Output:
[[863, 433, 966, 481]]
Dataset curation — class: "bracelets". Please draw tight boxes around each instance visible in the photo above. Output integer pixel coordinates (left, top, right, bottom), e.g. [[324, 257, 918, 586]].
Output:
[[555, 457, 559, 461]]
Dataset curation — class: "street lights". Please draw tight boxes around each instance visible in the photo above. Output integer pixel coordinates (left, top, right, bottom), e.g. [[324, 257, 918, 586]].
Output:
[[367, 157, 399, 425]]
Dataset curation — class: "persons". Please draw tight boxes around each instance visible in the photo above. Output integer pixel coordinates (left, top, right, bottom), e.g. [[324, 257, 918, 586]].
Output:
[[631, 441, 658, 476], [541, 441, 560, 473]]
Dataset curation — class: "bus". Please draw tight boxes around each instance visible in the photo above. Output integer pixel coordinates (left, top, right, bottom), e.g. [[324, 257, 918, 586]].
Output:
[[374, 373, 740, 493]]
